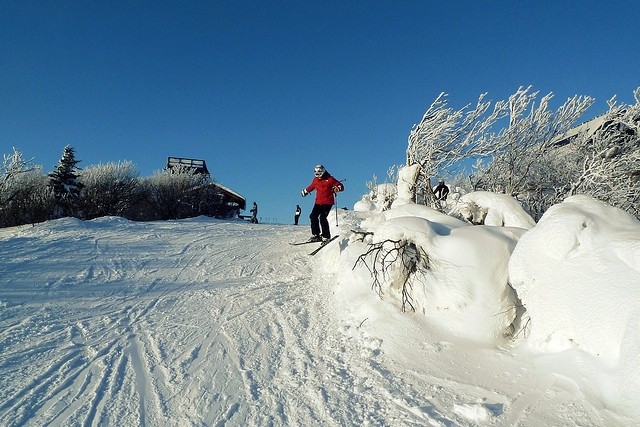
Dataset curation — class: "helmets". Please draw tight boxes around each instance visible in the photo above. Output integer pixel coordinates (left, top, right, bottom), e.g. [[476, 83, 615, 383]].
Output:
[[314, 165, 325, 179]]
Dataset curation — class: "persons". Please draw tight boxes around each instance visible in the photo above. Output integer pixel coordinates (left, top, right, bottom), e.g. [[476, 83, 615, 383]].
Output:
[[294, 204, 301, 225], [342, 206, 348, 210], [301, 164, 344, 243], [433, 178, 449, 201], [250, 201, 258, 224]]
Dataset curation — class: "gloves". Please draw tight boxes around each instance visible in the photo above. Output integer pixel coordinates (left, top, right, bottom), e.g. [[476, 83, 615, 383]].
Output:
[[332, 185, 341, 193], [301, 189, 310, 197]]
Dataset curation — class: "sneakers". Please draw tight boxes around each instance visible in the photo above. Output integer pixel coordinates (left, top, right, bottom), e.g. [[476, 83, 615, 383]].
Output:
[[322, 237, 331, 244], [309, 234, 320, 242]]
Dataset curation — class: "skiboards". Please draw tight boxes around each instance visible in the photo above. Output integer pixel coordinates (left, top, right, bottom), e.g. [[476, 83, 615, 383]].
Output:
[[289, 235, 340, 256]]
